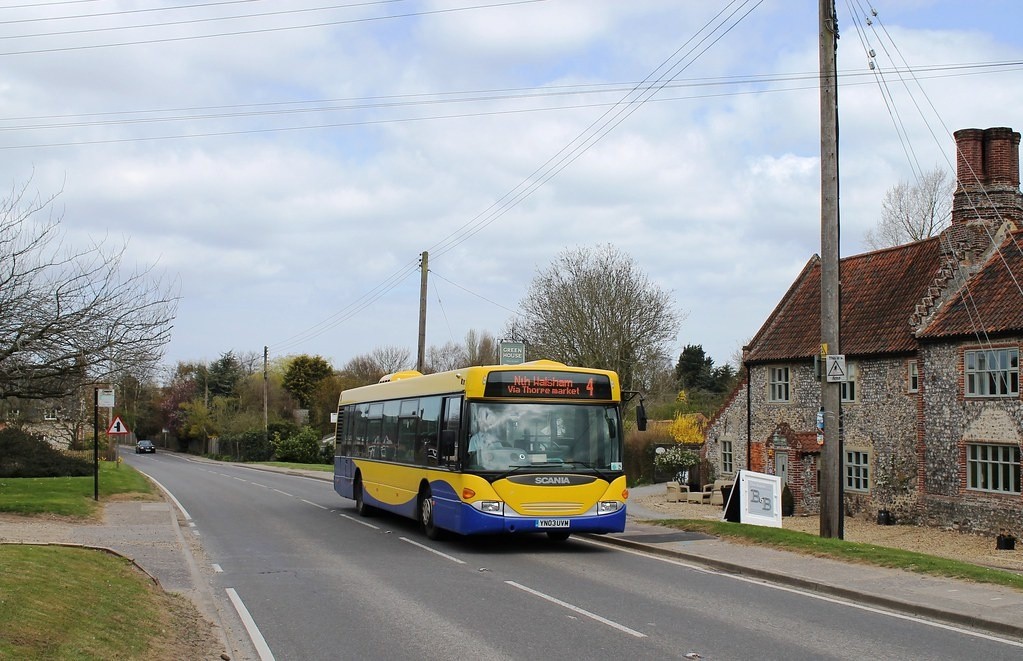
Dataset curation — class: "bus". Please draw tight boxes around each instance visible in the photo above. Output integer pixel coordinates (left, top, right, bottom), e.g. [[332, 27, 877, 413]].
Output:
[[333, 359, 649, 541]]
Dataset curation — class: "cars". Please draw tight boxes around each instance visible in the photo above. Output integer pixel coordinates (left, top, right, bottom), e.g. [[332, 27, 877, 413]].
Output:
[[135, 440, 156, 454]]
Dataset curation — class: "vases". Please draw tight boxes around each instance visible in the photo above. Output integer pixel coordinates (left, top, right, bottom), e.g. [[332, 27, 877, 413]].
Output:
[[997, 536, 1015, 550]]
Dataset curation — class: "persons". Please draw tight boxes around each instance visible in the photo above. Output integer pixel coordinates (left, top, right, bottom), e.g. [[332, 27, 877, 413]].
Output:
[[467, 417, 504, 452]]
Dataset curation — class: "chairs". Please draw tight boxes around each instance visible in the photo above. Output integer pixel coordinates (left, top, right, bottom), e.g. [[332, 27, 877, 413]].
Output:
[[419, 432, 526, 465]]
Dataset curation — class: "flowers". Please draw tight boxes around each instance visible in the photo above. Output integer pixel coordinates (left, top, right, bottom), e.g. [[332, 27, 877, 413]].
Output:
[[998, 531, 1021, 546]]
